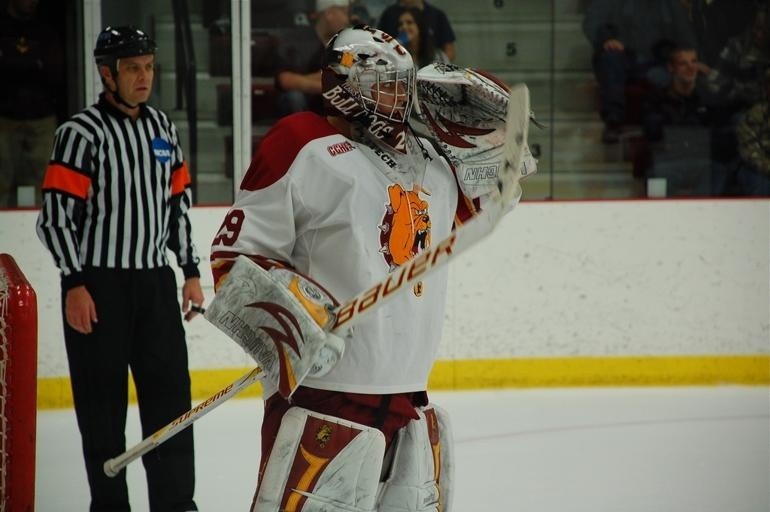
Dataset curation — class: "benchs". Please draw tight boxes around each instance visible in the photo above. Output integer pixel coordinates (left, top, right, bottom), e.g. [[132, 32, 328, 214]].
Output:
[[152, 1, 290, 201], [433, 3, 643, 198]]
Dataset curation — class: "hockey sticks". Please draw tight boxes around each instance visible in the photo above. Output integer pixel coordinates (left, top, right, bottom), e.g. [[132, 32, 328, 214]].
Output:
[[103, 82, 528, 477]]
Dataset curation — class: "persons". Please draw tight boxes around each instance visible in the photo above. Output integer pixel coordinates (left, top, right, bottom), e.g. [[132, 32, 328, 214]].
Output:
[[36, 26, 199, 512], [582, 0, 769, 197], [202, 27, 540, 512], [0, 0, 65, 207]]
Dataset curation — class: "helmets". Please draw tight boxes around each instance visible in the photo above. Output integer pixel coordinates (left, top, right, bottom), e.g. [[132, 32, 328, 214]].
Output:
[[318, 25, 416, 153], [93, 24, 159, 76]]
